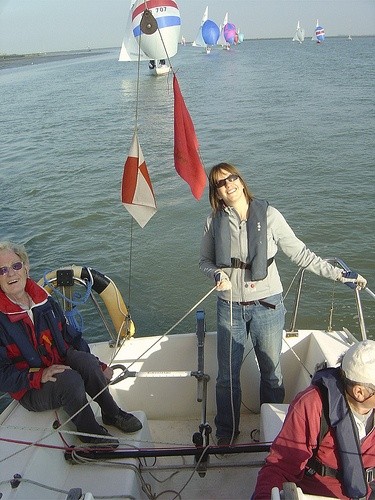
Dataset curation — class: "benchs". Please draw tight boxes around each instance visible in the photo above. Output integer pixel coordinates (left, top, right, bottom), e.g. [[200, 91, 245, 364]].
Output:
[[260, 403, 290, 444], [11, 398, 152, 500]]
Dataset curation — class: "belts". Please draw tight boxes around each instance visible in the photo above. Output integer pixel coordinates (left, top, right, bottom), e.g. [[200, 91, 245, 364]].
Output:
[[224, 297, 276, 309]]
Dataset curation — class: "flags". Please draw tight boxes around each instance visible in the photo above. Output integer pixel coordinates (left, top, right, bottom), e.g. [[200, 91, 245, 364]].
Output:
[[122, 132, 158, 230], [173, 73, 206, 199]]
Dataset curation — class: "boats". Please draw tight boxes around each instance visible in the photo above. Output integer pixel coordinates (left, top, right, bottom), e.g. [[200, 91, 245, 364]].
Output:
[[347, 34, 353, 42]]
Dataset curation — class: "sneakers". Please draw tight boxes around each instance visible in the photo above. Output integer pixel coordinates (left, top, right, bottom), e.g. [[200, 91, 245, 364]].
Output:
[[101, 408, 143, 432], [91, 426, 119, 449]]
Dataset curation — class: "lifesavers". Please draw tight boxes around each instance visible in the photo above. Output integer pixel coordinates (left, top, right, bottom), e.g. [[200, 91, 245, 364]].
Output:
[[34, 265, 136, 338]]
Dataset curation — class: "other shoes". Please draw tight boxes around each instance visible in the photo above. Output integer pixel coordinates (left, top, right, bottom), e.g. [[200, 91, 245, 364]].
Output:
[[216, 437, 234, 445]]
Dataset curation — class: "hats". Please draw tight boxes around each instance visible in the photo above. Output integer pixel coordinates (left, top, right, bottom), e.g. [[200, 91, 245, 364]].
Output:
[[342, 339, 375, 386]]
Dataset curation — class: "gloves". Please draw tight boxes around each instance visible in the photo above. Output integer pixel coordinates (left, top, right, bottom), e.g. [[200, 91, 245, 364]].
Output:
[[338, 270, 367, 290], [214, 271, 232, 291]]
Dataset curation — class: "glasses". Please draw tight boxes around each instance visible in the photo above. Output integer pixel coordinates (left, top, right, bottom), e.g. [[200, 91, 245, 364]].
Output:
[[0, 261, 24, 275], [215, 174, 238, 188]]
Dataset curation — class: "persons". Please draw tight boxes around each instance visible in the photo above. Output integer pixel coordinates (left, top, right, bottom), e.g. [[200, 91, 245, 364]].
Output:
[[251, 339, 375, 500], [0, 241, 142, 448], [198, 163, 368, 447]]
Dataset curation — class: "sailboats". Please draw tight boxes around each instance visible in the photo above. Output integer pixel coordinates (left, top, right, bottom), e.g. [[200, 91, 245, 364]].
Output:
[[118, 0, 182, 77], [291, 20, 305, 45], [216, 11, 244, 51], [180, 36, 187, 46], [311, 19, 326, 43], [192, 40, 198, 47], [191, 4, 221, 54]]
